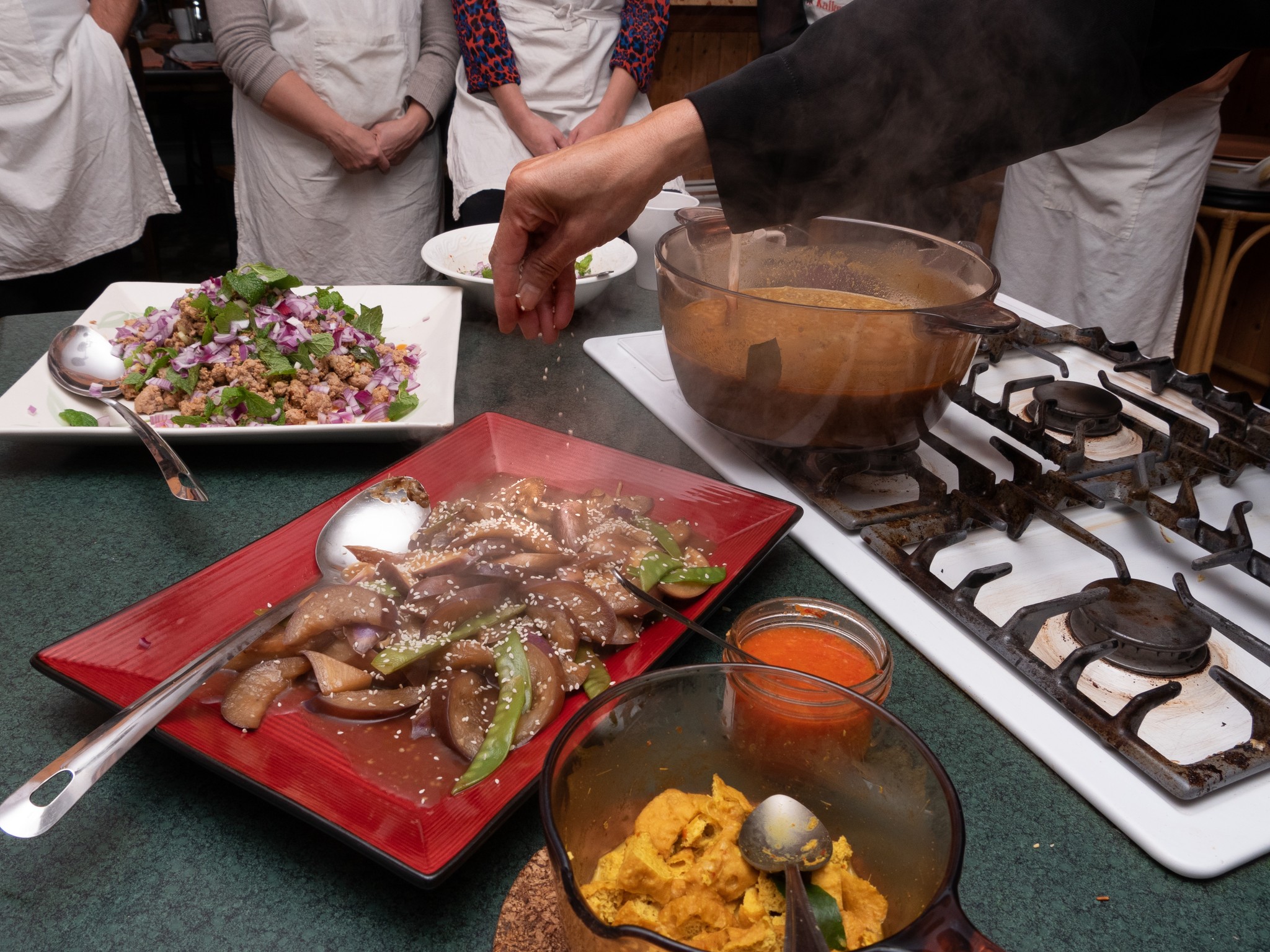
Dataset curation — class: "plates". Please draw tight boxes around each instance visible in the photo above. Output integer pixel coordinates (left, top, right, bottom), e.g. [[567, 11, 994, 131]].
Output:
[[28, 411, 806, 885], [0, 280, 463, 435]]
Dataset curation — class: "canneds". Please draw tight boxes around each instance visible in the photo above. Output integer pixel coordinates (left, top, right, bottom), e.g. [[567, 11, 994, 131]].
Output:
[[722, 596, 894, 786]]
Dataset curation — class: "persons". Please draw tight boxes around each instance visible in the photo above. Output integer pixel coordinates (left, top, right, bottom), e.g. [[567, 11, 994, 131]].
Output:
[[206, 1, 459, 283], [989, 53, 1248, 357], [492, 0, 1270, 345], [447, 0, 669, 243], [0, 0, 182, 313]]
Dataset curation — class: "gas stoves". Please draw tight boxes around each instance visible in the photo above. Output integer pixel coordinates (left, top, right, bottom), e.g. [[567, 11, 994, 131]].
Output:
[[582, 285, 1270, 881]]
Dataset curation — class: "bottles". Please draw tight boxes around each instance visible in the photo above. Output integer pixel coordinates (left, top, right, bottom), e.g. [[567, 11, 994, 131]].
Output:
[[720, 597, 896, 790], [168, 0, 213, 44]]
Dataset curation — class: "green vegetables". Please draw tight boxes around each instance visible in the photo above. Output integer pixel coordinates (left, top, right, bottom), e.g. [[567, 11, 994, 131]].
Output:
[[56, 262, 420, 428], [483, 254, 593, 279], [766, 868, 848, 952]]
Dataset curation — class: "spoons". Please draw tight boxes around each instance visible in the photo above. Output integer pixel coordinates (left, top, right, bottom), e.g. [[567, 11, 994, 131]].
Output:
[[0, 477, 431, 840], [737, 794, 833, 952], [46, 324, 209, 503]]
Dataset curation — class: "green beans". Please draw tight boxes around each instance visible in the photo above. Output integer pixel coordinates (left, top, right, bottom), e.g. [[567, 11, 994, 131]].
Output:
[[370, 520, 726, 793]]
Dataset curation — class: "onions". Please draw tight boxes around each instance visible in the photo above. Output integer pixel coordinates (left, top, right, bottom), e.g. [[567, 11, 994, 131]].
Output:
[[469, 261, 493, 278]]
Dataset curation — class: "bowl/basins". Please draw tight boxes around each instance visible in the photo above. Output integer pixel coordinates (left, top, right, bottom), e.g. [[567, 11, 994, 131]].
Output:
[[654, 206, 1021, 452], [420, 222, 638, 308], [537, 662, 1001, 952]]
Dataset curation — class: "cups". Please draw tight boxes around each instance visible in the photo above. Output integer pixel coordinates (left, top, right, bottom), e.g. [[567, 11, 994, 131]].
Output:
[[627, 191, 700, 291]]
